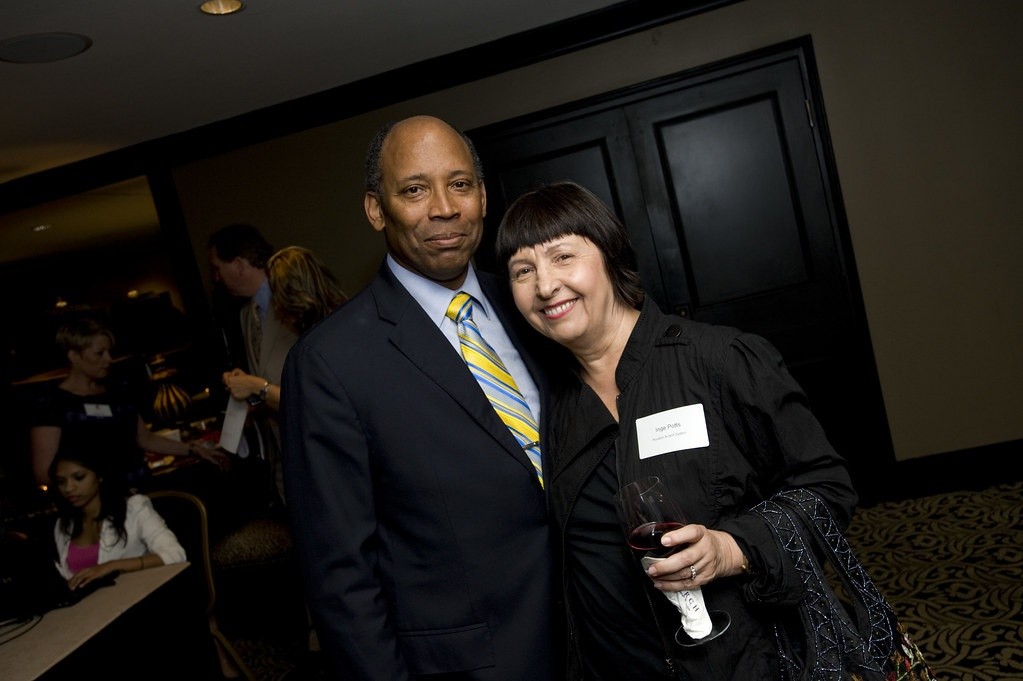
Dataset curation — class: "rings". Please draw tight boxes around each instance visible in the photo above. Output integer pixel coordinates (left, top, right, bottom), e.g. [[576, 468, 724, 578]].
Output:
[[690, 566, 696, 579]]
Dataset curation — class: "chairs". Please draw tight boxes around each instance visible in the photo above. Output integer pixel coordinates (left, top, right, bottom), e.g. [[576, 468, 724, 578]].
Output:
[[143, 489, 254, 681]]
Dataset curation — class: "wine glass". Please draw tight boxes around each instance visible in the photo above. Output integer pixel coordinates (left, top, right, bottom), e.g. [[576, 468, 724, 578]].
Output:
[[614, 477, 731, 647]]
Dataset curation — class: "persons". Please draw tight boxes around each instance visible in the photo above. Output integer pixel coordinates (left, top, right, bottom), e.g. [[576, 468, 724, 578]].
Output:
[[208, 223, 298, 412], [221, 245, 348, 410], [496, 180, 860, 681], [279, 114, 558, 681], [27, 318, 226, 497], [48, 441, 187, 590]]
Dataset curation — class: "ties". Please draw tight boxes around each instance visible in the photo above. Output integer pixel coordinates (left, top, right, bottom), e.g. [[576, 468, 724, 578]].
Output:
[[249, 302, 262, 366], [445, 292, 544, 490]]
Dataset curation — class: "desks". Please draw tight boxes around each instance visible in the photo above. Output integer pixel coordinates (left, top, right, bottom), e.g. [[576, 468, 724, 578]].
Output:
[[150, 429, 225, 479], [0, 561, 192, 681]]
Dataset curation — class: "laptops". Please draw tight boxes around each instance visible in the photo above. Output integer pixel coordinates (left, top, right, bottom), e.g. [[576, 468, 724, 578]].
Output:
[[0, 551, 120, 615]]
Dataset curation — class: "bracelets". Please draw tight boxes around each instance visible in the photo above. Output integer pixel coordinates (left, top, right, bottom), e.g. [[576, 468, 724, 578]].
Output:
[[189, 444, 194, 455], [140, 557, 144, 569], [742, 555, 751, 570]]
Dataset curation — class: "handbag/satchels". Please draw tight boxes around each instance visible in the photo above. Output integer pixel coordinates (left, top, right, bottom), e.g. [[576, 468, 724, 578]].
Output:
[[750, 489, 940, 681], [0, 530, 69, 621]]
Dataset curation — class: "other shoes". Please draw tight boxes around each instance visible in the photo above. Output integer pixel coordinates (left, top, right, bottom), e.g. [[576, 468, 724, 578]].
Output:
[[308, 629, 320, 651]]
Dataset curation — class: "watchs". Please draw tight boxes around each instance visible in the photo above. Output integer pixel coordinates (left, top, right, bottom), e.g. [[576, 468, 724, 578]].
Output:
[[250, 382, 269, 408]]
